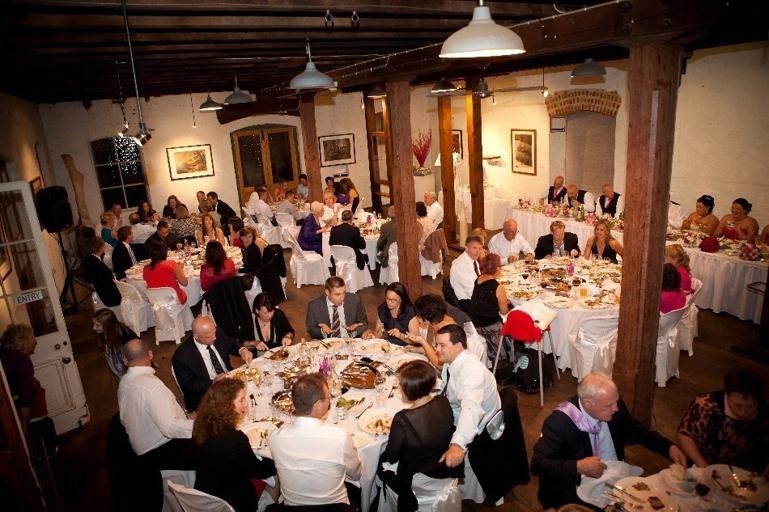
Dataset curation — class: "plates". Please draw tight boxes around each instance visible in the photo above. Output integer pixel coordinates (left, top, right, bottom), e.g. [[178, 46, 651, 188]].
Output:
[[612, 467, 768, 512], [225, 337, 429, 450], [124, 258, 151, 281], [507, 258, 618, 310]]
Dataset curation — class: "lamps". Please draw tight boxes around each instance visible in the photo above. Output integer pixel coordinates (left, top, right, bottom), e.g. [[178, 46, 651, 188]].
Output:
[[288, 39, 338, 92], [438, 2, 527, 59], [120, 1, 152, 148], [198, 77, 253, 112]]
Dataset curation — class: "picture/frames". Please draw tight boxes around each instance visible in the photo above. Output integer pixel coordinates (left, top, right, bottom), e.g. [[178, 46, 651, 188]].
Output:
[[317, 132, 357, 168], [451, 128, 463, 160], [510, 128, 537, 176], [30, 176, 49, 233], [165, 143, 215, 182]]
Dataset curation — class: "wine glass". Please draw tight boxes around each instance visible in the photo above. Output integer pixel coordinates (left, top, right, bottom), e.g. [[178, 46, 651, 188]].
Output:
[[250, 331, 390, 434], [354, 210, 382, 224], [512, 253, 612, 293]]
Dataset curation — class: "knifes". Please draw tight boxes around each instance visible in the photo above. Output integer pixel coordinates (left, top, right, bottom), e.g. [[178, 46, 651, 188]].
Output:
[[728, 464, 741, 487], [604, 482, 644, 504]]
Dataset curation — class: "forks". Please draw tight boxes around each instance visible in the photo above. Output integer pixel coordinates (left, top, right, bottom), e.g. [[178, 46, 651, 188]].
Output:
[[601, 491, 643, 511]]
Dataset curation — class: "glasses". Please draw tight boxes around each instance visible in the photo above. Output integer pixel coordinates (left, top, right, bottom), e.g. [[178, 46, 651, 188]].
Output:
[[507, 230, 519, 233], [383, 296, 396, 303]]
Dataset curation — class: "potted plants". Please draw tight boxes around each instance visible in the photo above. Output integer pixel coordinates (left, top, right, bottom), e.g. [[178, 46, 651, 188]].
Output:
[[412, 127, 433, 177]]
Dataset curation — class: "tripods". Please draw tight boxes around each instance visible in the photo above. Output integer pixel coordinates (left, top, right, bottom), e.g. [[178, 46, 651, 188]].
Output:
[[56, 231, 94, 311]]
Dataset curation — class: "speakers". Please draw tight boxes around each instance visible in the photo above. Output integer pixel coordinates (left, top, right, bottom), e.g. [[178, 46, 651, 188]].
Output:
[[33, 186, 74, 234]]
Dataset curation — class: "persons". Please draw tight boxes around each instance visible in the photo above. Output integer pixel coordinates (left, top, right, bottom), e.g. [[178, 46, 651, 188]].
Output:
[[76, 190, 270, 306], [116, 277, 501, 511], [542, 177, 769, 244], [253, 174, 368, 267], [377, 192, 444, 259], [91, 310, 138, 381], [448, 219, 692, 358], [529, 370, 769, 509], [0, 322, 39, 438]]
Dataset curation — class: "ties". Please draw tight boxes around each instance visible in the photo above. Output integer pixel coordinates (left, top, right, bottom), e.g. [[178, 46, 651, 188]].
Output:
[[553, 190, 557, 199], [206, 345, 224, 374], [439, 367, 449, 396], [473, 261, 479, 278], [331, 305, 340, 338], [553, 401, 601, 456], [128, 247, 137, 264], [606, 198, 611, 207]]
